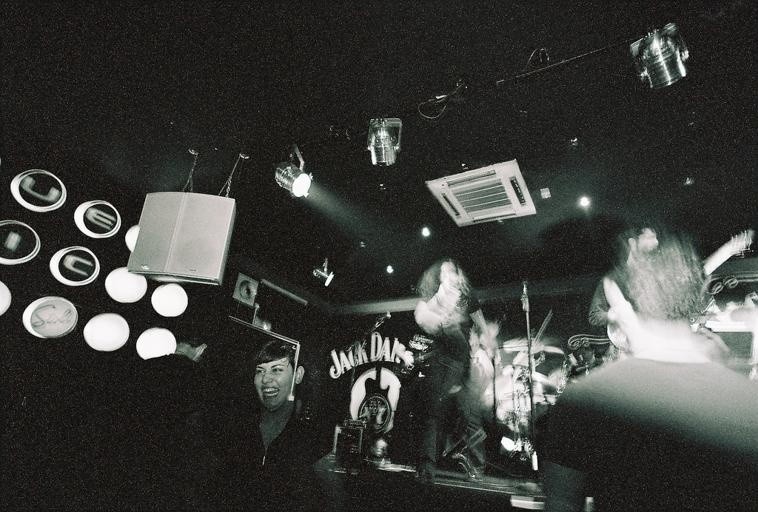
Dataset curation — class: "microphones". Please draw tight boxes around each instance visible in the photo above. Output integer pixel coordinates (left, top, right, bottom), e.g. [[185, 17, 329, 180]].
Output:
[[376, 311, 392, 321], [521, 281, 530, 312]]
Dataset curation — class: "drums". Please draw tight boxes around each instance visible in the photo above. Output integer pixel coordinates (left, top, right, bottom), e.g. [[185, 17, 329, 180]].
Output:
[[349, 363, 403, 433]]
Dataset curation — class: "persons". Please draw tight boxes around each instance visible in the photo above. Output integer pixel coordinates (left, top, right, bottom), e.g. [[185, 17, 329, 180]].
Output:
[[369, 224, 757, 511], [212, 340, 318, 509]]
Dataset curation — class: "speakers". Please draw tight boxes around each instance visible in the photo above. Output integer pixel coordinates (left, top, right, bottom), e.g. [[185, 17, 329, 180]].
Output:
[[166, 191, 238, 287], [126, 192, 183, 283]]
